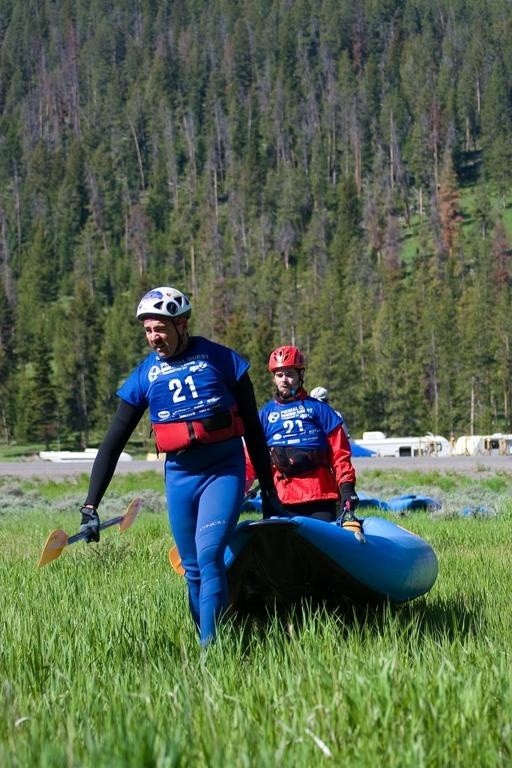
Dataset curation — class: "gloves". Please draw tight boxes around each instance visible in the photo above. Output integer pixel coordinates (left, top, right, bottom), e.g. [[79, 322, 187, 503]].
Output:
[[339, 482, 359, 512], [78, 506, 100, 544]]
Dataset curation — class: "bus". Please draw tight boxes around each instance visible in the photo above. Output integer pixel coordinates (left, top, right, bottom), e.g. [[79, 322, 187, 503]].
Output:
[[39, 447, 132, 463], [353, 431, 512, 458]]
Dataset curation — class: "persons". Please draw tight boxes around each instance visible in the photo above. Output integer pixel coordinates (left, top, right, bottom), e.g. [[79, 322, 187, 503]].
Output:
[[312, 385, 350, 440], [244, 344, 359, 519], [81, 285, 290, 651]]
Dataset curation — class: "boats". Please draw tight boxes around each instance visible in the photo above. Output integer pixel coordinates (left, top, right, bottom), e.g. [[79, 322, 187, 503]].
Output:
[[223, 514, 439, 622]]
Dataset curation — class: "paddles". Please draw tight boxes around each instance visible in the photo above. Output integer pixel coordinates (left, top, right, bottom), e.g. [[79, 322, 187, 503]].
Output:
[[37, 496, 141, 569], [168, 483, 261, 577]]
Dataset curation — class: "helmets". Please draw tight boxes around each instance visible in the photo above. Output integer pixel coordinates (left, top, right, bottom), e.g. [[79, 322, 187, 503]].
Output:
[[136, 286, 192, 320], [268, 345, 306, 373]]
